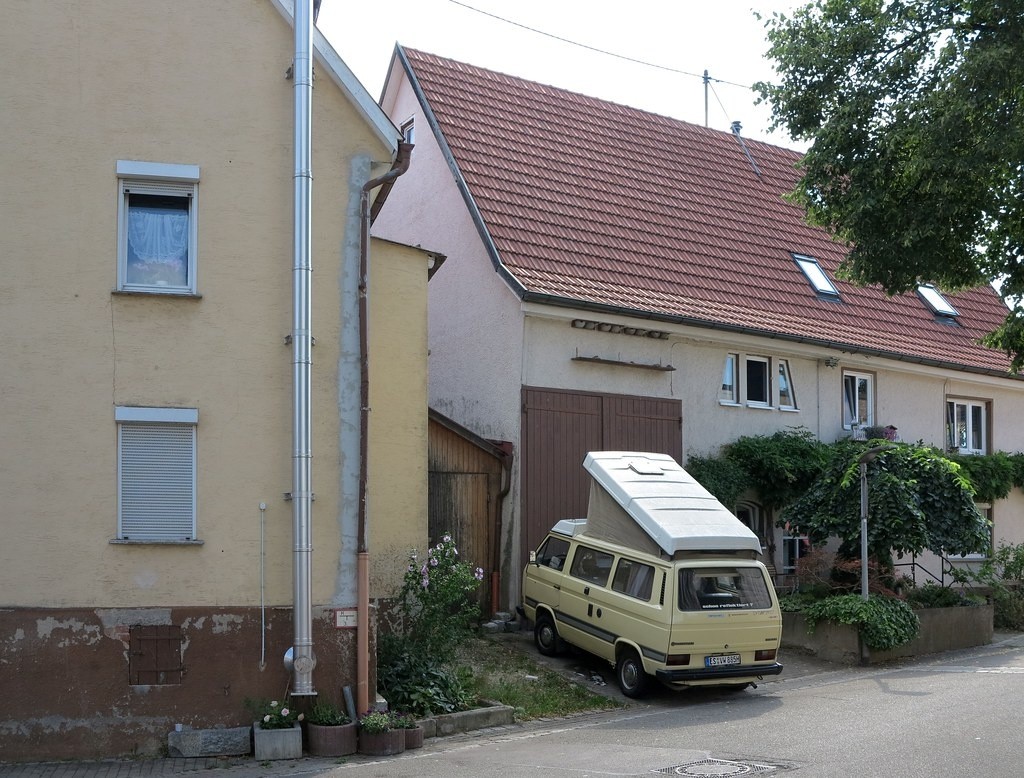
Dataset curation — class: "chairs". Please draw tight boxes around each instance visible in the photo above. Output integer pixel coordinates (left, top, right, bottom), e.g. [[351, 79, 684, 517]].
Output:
[[699, 593, 732, 609], [578, 551, 600, 577]]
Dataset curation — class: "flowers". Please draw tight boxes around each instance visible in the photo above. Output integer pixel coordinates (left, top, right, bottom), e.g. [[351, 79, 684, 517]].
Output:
[[860, 425, 897, 441], [354, 708, 408, 737], [258, 700, 304, 729]]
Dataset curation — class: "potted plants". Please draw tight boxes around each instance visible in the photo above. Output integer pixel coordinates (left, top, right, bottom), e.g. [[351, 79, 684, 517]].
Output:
[[405, 713, 424, 749], [305, 701, 357, 756]]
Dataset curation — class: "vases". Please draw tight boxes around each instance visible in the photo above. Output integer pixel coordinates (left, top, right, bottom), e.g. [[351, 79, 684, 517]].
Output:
[[359, 729, 405, 756], [253, 722, 302, 761], [864, 432, 883, 440]]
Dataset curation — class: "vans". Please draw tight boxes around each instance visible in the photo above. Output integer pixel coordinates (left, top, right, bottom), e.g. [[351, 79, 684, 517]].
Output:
[[521, 448, 785, 696]]
